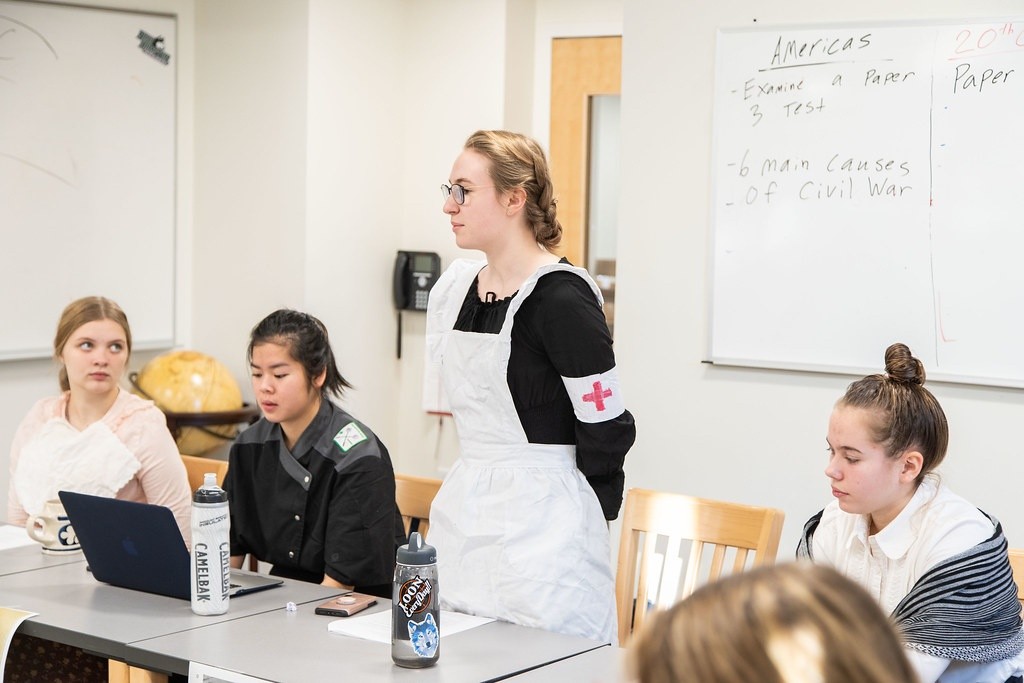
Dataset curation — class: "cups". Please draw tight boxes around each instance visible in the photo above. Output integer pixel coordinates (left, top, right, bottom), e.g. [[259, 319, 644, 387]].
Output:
[[26, 498, 81, 550], [391, 532, 441, 668], [190, 472, 231, 616]]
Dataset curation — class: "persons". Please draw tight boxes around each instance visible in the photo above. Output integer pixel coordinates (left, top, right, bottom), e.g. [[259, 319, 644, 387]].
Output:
[[796, 342, 1024, 683], [222, 309, 407, 598], [422, 130, 637, 649], [7, 295, 196, 553], [628, 563, 921, 683]]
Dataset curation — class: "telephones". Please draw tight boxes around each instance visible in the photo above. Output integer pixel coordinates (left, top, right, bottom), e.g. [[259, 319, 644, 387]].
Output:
[[392, 249, 442, 314]]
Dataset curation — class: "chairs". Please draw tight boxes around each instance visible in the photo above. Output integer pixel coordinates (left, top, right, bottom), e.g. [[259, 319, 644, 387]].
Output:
[[106, 455, 787, 682]]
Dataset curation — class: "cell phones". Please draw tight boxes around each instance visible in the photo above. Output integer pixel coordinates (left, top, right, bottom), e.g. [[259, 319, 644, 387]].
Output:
[[314, 593, 377, 618]]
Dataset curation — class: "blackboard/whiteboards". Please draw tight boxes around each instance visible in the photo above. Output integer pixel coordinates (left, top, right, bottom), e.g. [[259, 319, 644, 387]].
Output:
[[700, 8, 1024, 394]]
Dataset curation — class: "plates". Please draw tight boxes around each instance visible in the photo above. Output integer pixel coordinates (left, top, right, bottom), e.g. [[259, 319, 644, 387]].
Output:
[[41, 546, 82, 556]]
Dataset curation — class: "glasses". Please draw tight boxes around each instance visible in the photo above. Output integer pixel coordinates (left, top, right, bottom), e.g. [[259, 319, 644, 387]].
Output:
[[440, 183, 496, 205]]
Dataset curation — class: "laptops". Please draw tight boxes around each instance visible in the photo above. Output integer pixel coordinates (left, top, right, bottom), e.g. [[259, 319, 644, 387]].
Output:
[[58, 490, 285, 606]]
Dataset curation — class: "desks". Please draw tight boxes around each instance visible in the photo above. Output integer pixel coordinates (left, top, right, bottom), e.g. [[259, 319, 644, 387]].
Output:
[[0, 521, 629, 683]]
